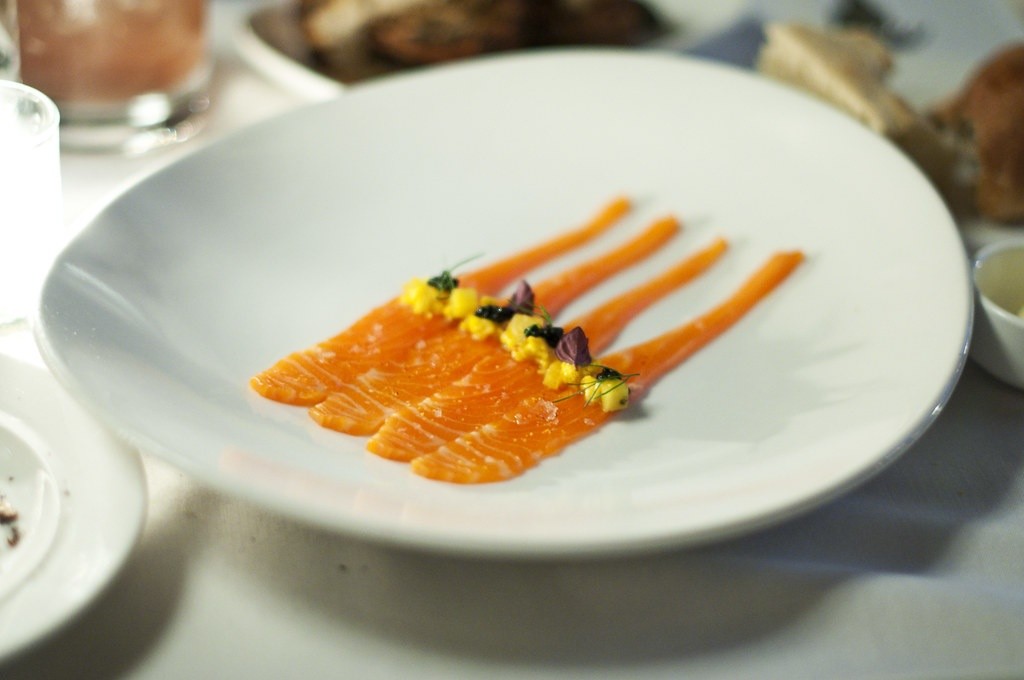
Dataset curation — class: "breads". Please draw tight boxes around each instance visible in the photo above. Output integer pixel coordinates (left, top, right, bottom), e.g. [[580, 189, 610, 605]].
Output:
[[296, 1, 673, 68], [754, 24, 1023, 223]]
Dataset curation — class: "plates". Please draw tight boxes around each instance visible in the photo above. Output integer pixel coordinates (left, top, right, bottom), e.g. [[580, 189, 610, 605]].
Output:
[[33, 47, 977, 567], [209, 0, 756, 101], [0, 350, 148, 663]]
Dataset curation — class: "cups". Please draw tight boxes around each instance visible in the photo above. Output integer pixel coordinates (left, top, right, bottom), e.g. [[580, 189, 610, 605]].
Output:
[[1, 78, 66, 329], [0, 1, 211, 146], [972, 236, 1024, 389]]
